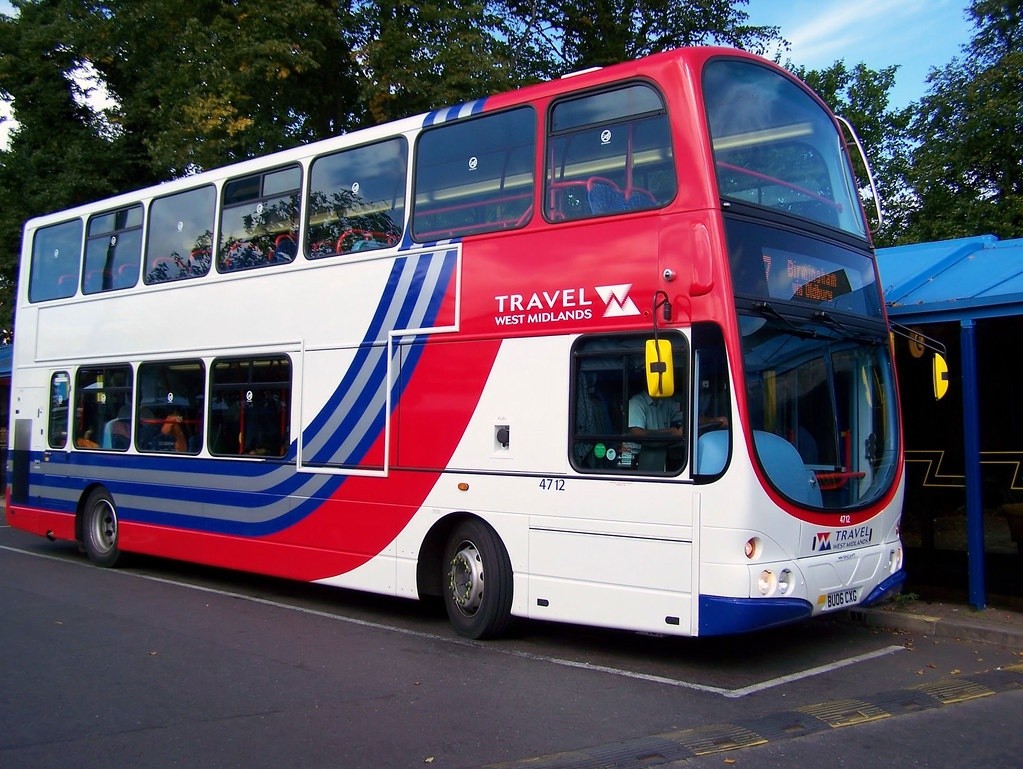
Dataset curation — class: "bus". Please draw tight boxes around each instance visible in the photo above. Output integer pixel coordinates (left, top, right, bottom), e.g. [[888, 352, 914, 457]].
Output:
[[0, 45, 952, 641]]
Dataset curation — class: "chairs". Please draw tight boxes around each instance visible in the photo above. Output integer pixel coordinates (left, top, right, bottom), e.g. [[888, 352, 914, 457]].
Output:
[[104, 417, 129, 449], [30, 176, 662, 303]]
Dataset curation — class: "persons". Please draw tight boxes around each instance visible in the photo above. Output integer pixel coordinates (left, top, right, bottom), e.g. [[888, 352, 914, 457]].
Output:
[[160, 399, 191, 452], [627, 370, 729, 472], [113, 403, 131, 448]]
